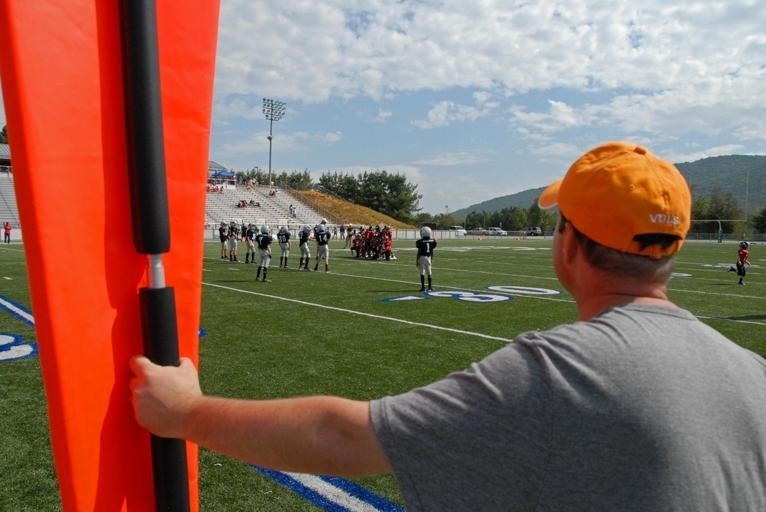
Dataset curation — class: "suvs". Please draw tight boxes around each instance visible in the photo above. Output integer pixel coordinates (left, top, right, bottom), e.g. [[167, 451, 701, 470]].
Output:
[[449, 226, 467, 235]]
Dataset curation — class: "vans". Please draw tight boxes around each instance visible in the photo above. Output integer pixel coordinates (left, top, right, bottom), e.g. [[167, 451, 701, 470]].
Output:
[[527, 227, 543, 236]]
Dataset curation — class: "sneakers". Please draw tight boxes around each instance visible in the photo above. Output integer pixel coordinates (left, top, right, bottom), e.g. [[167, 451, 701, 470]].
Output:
[[419, 287, 435, 291], [728, 266, 733, 271], [221, 256, 329, 272], [739, 282, 745, 286]]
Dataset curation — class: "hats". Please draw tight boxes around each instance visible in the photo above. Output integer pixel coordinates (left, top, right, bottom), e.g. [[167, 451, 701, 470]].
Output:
[[539, 143, 692, 255], [256, 277, 272, 282]]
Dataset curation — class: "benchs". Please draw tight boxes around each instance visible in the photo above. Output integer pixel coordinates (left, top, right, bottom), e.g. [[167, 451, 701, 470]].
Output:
[[0, 174, 21, 232], [202, 185, 330, 227]]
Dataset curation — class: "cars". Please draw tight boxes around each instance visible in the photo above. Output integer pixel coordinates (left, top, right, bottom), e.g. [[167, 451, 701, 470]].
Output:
[[471, 226, 507, 236]]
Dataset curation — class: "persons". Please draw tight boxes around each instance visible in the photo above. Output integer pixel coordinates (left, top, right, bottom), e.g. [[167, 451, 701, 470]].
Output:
[[127, 140, 766, 512], [3, 221, 11, 244], [413, 225, 437, 292], [206, 175, 397, 283], [728, 241, 751, 286]]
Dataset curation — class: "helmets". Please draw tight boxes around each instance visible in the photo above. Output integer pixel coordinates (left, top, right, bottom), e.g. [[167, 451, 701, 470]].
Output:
[[420, 227, 432, 238], [220, 221, 328, 235], [740, 242, 750, 249]]
